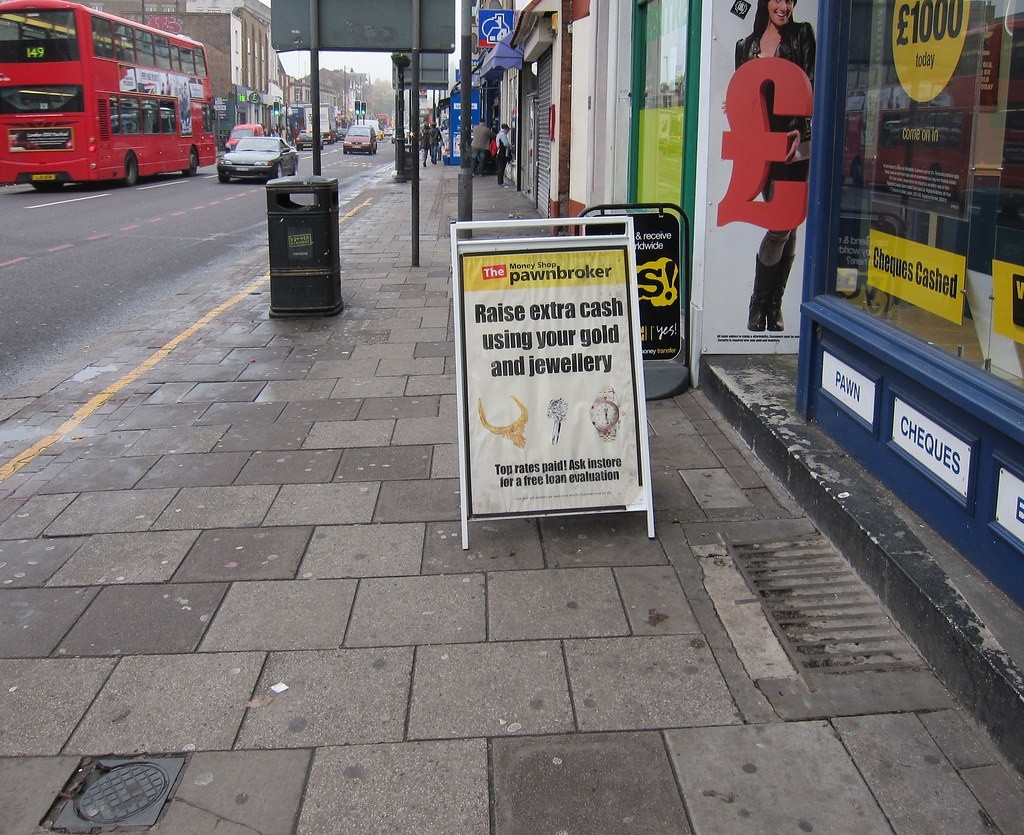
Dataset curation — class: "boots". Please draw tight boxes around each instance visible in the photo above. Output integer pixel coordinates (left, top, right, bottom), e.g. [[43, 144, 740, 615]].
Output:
[[747, 253, 796, 332]]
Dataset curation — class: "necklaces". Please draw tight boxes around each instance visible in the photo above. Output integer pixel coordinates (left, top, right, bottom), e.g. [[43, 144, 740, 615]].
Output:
[[478, 395, 529, 451]]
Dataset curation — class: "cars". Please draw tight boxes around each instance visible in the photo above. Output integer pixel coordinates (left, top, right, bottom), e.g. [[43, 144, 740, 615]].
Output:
[[383, 127, 393, 136], [218, 137, 298, 183], [336, 128, 348, 141], [296, 130, 323, 151]]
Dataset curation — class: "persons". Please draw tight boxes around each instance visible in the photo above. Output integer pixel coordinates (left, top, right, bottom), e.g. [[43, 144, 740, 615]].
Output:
[[495, 123, 516, 185], [470, 118, 498, 177], [420, 118, 450, 167], [256, 123, 307, 147], [722, 0, 817, 332]]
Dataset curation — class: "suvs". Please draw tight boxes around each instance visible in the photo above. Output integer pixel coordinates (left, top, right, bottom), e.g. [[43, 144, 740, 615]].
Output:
[[392, 129, 409, 143]]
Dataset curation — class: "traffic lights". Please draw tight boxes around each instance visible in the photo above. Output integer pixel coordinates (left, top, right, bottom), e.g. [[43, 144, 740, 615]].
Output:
[[274, 101, 280, 115], [354, 100, 361, 116], [361, 102, 366, 115]]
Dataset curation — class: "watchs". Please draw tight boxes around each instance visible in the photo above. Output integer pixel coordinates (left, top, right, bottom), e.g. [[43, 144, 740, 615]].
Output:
[[588, 386, 621, 443]]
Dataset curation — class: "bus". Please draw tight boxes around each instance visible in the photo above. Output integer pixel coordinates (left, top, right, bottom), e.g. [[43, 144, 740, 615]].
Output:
[[375, 114, 389, 128], [840, 19, 1024, 200], [0, 0, 216, 189]]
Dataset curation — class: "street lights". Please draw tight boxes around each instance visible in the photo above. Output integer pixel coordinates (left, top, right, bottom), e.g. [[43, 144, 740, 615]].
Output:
[[343, 65, 357, 125], [360, 77, 368, 100]]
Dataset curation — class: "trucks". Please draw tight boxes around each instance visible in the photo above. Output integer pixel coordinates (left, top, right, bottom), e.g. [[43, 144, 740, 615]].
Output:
[[303, 103, 338, 145], [355, 119, 383, 141]]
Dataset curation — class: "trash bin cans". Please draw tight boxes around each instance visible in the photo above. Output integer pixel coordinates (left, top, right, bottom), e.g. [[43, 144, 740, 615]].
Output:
[[403, 144, 412, 182], [265, 176, 344, 319]]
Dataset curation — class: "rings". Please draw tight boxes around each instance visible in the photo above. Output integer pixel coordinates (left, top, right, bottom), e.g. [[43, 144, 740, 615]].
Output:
[[548, 398, 569, 446]]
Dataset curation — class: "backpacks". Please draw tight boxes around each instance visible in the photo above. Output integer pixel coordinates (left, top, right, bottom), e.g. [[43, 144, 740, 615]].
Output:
[[490, 136, 500, 156]]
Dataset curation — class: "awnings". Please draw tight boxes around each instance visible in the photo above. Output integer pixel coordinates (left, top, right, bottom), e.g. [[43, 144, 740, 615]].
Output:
[[480, 29, 525, 82]]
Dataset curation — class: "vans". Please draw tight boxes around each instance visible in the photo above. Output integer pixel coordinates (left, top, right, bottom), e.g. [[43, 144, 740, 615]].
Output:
[[226, 124, 264, 152], [343, 125, 378, 155]]
